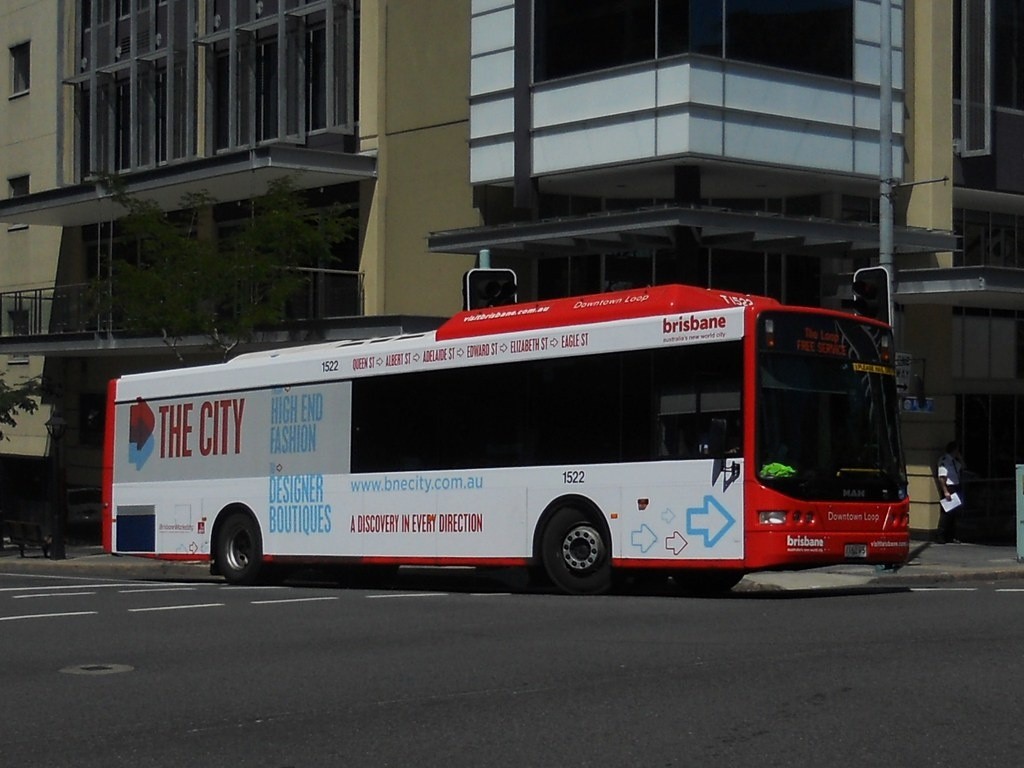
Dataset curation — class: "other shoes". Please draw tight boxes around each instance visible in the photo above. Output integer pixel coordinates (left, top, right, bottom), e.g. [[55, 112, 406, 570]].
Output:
[[946, 537, 961, 543], [936, 537, 946, 544]]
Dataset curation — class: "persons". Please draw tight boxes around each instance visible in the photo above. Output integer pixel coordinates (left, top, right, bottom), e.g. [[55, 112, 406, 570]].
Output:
[[934, 440, 966, 544]]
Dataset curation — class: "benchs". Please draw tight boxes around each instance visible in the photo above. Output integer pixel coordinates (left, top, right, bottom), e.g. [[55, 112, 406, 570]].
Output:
[[3, 520, 68, 559]]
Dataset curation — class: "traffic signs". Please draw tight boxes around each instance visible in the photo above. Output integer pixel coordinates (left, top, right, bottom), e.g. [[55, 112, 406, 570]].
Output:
[[894, 351, 912, 394]]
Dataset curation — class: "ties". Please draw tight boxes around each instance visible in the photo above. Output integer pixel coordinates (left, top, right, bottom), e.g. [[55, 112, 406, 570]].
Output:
[[951, 459, 961, 483]]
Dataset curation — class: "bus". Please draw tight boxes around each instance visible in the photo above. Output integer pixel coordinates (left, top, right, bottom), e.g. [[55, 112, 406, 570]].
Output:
[[101, 283, 927, 595]]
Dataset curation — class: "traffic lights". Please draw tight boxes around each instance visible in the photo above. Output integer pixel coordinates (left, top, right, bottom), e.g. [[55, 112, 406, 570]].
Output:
[[850, 265, 889, 321], [463, 269, 518, 311]]
